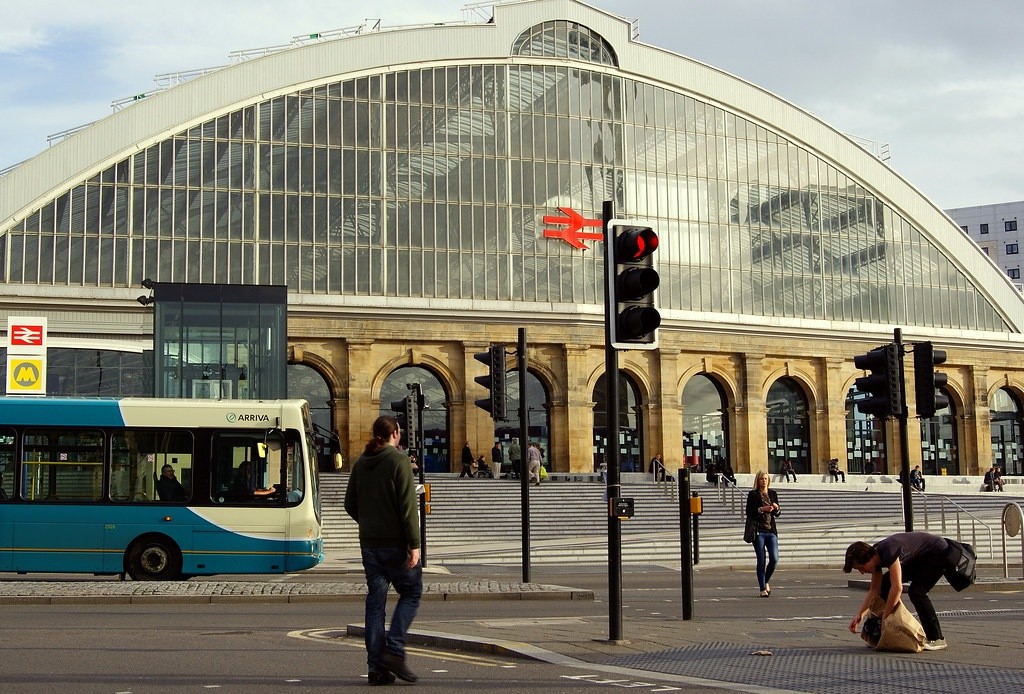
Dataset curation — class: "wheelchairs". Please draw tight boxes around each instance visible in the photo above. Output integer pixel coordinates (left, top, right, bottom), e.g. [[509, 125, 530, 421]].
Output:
[[472, 459, 492, 479]]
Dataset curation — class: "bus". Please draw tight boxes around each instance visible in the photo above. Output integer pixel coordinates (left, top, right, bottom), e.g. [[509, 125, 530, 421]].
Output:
[[0, 396, 344, 581]]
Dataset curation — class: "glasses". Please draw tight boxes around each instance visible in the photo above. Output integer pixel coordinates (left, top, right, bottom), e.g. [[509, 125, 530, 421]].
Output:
[[397, 429, 406, 435]]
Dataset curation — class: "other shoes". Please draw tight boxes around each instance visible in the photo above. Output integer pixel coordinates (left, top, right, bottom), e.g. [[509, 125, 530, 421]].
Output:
[[535, 482, 540, 486], [367, 669, 396, 685], [378, 649, 417, 683], [766, 589, 771, 595], [760, 593, 769, 598]]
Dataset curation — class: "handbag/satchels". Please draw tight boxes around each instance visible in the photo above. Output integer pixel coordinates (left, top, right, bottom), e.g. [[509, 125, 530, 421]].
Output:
[[943, 538, 978, 592], [860, 596, 926, 653], [743, 518, 757, 543], [540, 465, 548, 480]]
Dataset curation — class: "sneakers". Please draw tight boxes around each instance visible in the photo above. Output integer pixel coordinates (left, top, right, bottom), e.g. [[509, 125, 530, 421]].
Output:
[[924, 638, 948, 650]]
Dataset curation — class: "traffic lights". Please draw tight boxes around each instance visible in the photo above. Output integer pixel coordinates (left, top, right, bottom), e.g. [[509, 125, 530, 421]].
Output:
[[854, 345, 890, 421], [914, 343, 949, 420], [391, 397, 416, 450], [474, 344, 508, 422], [607, 220, 662, 349]]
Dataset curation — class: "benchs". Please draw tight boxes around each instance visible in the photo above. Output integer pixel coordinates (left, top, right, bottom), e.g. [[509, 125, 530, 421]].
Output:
[[478, 470, 487, 478], [533, 471, 600, 483]]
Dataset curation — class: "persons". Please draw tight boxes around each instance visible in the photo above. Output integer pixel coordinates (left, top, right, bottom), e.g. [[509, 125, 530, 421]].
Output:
[[722, 467, 737, 487], [159, 464, 187, 501], [828, 458, 847, 483], [235, 461, 276, 503], [706, 464, 720, 487], [408, 454, 419, 477], [344, 415, 423, 685], [984, 467, 1007, 492], [843, 532, 949, 650], [459, 440, 476, 478], [746, 470, 781, 598], [528, 442, 545, 485], [648, 454, 665, 482], [508, 439, 521, 479], [900, 465, 925, 492], [781, 458, 798, 483], [478, 455, 493, 478], [492, 442, 502, 480]]
